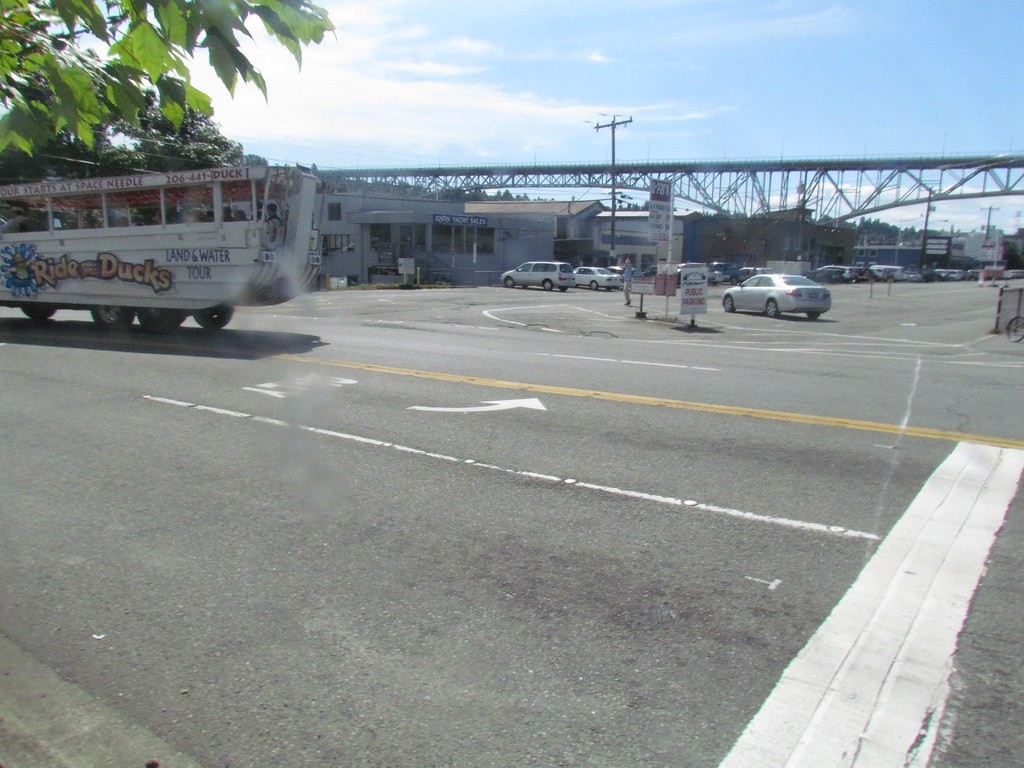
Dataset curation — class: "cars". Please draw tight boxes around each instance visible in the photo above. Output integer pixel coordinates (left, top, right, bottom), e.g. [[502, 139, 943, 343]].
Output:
[[500, 261, 577, 291], [608, 260, 1024, 287], [573, 267, 622, 291], [721, 273, 831, 319]]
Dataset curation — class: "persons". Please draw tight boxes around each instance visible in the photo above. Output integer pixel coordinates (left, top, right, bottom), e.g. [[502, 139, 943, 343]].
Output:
[[621, 256, 634, 305], [749, 266, 757, 277], [5, 198, 282, 235]]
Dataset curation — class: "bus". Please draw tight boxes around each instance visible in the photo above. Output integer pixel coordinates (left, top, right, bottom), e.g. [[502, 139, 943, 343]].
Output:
[[1, 165, 327, 332]]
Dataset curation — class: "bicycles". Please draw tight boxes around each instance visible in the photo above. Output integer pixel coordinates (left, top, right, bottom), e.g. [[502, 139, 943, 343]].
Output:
[[1004, 315, 1024, 343]]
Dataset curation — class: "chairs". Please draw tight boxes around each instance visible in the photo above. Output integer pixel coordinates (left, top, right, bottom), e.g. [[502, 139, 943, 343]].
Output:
[[8, 205, 256, 233]]
